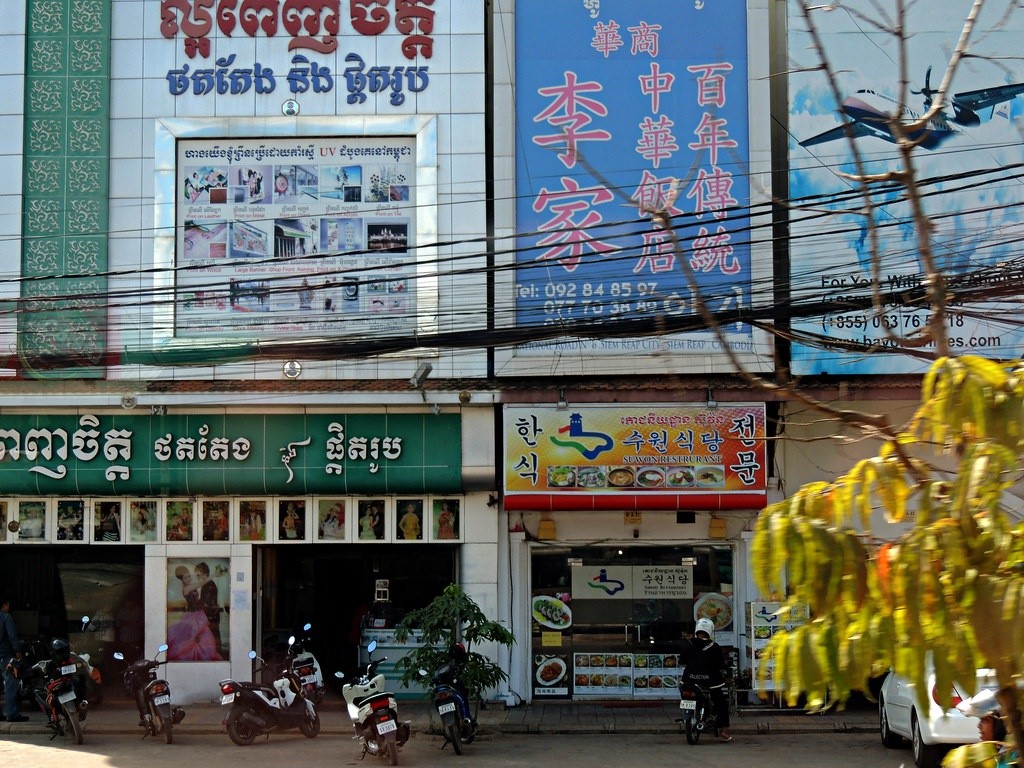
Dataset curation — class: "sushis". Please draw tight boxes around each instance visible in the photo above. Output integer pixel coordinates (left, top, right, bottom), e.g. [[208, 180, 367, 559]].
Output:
[[537, 600, 564, 624]]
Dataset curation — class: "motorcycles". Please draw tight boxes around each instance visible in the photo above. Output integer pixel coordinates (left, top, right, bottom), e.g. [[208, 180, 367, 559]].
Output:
[[47, 615, 105, 707], [1, 636, 56, 721], [333, 638, 411, 764], [113, 644, 185, 745], [280, 623, 327, 711], [220, 650, 320, 745], [9, 639, 88, 745], [418, 642, 479, 756], [673, 652, 735, 745]]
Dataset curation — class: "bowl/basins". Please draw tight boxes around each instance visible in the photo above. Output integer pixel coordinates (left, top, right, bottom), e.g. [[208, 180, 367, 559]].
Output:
[[664, 656, 676, 668], [635, 678, 648, 688], [756, 629, 771, 638], [662, 676, 678, 688], [755, 667, 771, 680], [536, 658, 566, 686], [694, 592, 733, 630]]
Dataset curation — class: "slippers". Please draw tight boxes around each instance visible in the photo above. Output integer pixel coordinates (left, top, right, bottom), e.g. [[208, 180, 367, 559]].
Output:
[[720, 736, 736, 743]]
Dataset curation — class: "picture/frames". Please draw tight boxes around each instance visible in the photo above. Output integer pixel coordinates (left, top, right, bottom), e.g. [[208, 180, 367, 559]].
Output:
[[144, 545, 252, 704], [14, 498, 52, 544], [353, 496, 391, 543], [429, 496, 465, 543], [125, 498, 161, 545], [197, 498, 233, 543], [312, 497, 351, 543], [0, 498, 13, 543], [273, 497, 312, 543], [392, 496, 428, 543], [52, 498, 89, 544], [161, 498, 197, 545], [234, 497, 274, 543], [90, 498, 125, 545]]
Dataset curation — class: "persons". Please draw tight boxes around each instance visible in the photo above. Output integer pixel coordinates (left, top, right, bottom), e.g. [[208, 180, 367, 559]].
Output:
[[955, 685, 1024, 768], [166, 563, 229, 662], [0, 501, 457, 542], [0, 594, 31, 723], [677, 618, 735, 743]]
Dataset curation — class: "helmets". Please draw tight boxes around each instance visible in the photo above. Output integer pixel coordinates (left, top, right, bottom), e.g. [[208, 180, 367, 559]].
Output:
[[694, 617, 716, 640]]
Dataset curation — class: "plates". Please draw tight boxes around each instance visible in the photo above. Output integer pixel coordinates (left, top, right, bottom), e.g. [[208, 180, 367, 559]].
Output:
[[532, 596, 572, 629]]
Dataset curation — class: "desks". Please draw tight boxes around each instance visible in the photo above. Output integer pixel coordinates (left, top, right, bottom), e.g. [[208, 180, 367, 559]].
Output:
[[358, 630, 450, 701]]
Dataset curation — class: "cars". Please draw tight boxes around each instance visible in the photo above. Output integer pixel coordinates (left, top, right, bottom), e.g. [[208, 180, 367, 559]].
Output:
[[879, 648, 1002, 768]]
